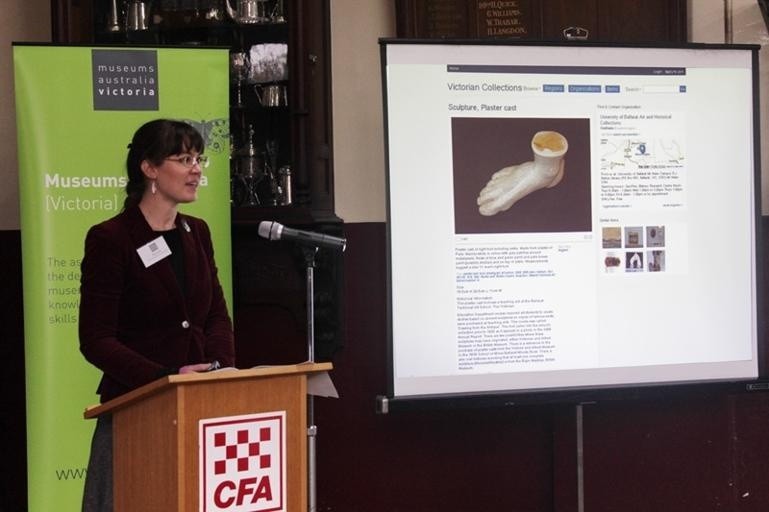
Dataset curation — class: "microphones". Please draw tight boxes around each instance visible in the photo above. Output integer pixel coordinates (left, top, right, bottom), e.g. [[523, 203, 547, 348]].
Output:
[[258, 220, 347, 253]]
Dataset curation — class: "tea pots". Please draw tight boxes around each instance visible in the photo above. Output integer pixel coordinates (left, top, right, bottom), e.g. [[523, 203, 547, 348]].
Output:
[[116, 1, 160, 33], [252, 79, 288, 109], [264, 161, 303, 206], [224, 1, 269, 25]]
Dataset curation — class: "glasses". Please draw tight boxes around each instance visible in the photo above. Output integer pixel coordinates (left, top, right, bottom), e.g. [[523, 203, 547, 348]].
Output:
[[163, 155, 211, 168]]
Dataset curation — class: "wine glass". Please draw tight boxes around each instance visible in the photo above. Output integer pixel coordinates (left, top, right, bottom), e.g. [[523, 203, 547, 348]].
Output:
[[235, 122, 268, 209]]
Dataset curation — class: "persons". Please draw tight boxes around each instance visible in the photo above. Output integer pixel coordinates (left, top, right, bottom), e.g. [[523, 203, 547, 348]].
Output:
[[77, 118, 236, 511], [475, 130, 569, 217]]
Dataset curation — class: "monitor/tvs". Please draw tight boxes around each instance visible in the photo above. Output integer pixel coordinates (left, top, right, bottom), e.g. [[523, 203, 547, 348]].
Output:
[[247, 42, 290, 86]]
